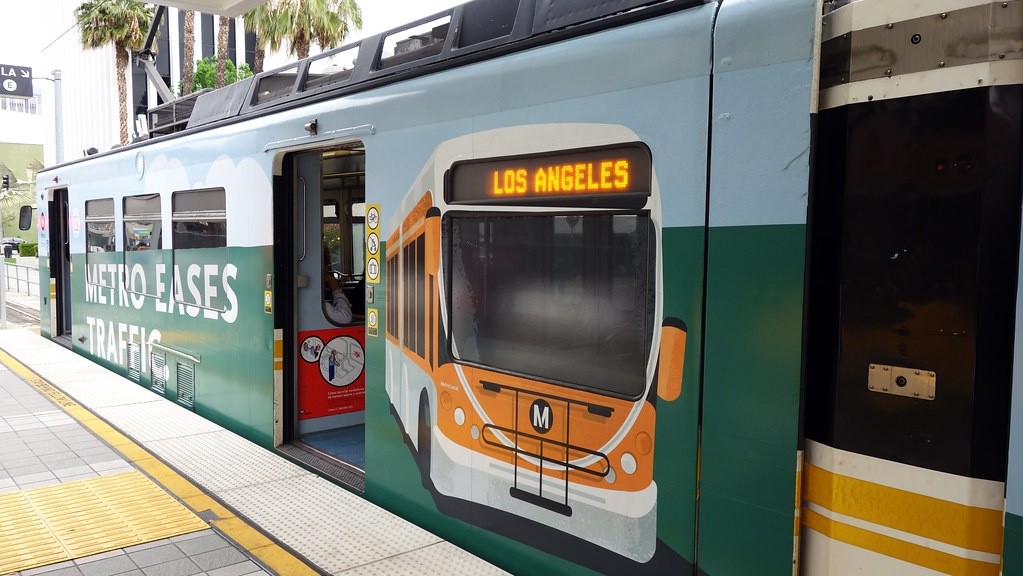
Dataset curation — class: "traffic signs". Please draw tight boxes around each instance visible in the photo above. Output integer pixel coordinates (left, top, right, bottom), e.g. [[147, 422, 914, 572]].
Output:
[[0, 64, 33, 99]]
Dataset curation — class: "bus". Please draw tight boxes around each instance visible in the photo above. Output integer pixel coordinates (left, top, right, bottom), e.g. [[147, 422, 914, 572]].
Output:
[[19, 0, 1023, 576]]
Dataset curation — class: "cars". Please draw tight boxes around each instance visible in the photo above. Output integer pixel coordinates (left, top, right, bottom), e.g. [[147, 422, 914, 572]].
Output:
[[1, 237, 27, 252]]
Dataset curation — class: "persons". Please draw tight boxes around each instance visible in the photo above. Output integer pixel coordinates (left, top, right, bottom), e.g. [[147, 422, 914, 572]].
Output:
[[320, 273, 353, 325]]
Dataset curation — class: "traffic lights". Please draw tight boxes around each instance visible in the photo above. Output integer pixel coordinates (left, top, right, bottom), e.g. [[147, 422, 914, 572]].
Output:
[[3, 175, 9, 189]]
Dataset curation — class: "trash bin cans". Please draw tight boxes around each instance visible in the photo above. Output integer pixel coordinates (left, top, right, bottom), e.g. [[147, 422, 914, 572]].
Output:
[[4, 245, 12, 258]]
